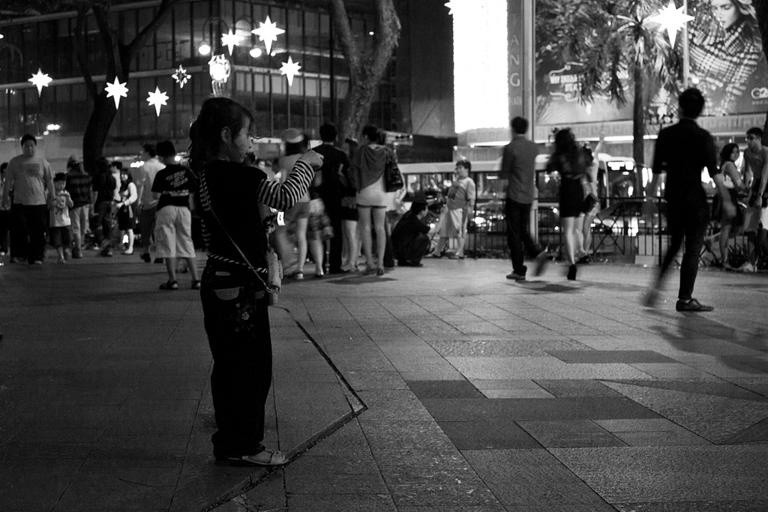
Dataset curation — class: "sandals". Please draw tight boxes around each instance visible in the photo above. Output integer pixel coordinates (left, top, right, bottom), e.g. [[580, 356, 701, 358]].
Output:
[[220, 445, 291, 467], [191, 279, 203, 290], [432, 248, 465, 260], [159, 280, 179, 290]]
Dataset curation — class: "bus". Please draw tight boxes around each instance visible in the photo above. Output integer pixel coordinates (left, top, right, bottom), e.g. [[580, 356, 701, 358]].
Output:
[[395, 152, 654, 244]]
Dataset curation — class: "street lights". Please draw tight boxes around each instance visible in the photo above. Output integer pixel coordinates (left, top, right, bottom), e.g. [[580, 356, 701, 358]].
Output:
[[196, 17, 263, 107]]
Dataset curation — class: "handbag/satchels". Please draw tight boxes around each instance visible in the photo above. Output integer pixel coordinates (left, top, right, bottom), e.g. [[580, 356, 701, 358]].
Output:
[[580, 193, 598, 215], [384, 145, 404, 193], [267, 288, 279, 305], [713, 198, 722, 217]]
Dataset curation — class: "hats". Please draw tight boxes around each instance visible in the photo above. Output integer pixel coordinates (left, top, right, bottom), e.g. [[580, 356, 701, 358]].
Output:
[[281, 127, 305, 144]]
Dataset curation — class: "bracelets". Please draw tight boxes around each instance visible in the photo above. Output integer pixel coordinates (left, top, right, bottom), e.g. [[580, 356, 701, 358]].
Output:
[[758, 192, 762, 196]]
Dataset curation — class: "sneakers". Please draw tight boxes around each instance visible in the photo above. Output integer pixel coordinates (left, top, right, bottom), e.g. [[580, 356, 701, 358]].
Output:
[[506, 271, 526, 281], [0, 243, 135, 266], [575, 253, 594, 265], [567, 263, 577, 280]]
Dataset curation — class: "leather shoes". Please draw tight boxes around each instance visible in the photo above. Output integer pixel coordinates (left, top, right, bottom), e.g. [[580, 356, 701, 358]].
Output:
[[676, 298, 714, 312], [140, 253, 165, 264], [283, 263, 386, 281], [397, 259, 425, 267]]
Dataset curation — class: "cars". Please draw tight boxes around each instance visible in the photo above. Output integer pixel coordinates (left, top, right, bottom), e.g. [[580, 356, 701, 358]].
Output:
[[410, 198, 676, 238]]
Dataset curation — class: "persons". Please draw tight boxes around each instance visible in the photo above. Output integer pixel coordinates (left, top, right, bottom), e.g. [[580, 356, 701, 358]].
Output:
[[391, 199, 433, 267], [710, 144, 749, 270], [1, 121, 406, 292], [643, 88, 737, 311], [580, 140, 603, 264], [432, 160, 477, 260], [189, 98, 325, 466], [655, 0, 762, 117], [547, 129, 595, 281], [498, 117, 546, 280], [738, 128, 768, 273]]
